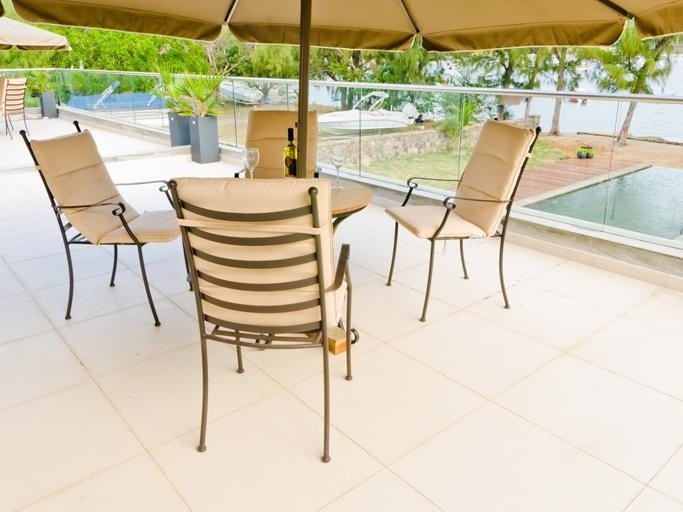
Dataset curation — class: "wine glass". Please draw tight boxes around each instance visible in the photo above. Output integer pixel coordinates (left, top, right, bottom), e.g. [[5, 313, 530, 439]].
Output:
[[328, 145, 347, 191], [242, 148, 260, 179]]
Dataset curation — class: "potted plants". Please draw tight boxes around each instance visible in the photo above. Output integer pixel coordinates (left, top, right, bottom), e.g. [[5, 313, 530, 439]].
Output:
[[577, 145, 594, 159], [154, 58, 233, 165], [27, 70, 57, 117]]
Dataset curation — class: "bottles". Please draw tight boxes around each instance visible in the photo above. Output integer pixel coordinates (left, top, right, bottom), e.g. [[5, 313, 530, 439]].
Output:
[[283, 128, 297, 179]]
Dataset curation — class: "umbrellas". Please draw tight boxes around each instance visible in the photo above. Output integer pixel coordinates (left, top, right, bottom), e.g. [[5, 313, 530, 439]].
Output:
[[10, 0, 683, 178], [0, 15, 73, 52]]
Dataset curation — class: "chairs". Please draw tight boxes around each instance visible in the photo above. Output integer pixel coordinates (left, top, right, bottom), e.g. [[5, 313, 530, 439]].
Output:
[[159, 178, 352, 463], [233, 106, 321, 178], [386, 118, 543, 323], [18, 121, 193, 326], [0, 76, 31, 138]]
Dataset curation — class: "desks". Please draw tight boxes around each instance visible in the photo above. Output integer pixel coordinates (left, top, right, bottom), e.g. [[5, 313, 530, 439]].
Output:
[[329, 176, 371, 346]]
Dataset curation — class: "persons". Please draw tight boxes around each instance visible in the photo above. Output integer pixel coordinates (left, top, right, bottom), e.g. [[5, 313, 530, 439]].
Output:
[[568, 97, 587, 105], [415, 114, 424, 123], [494, 104, 505, 121]]
